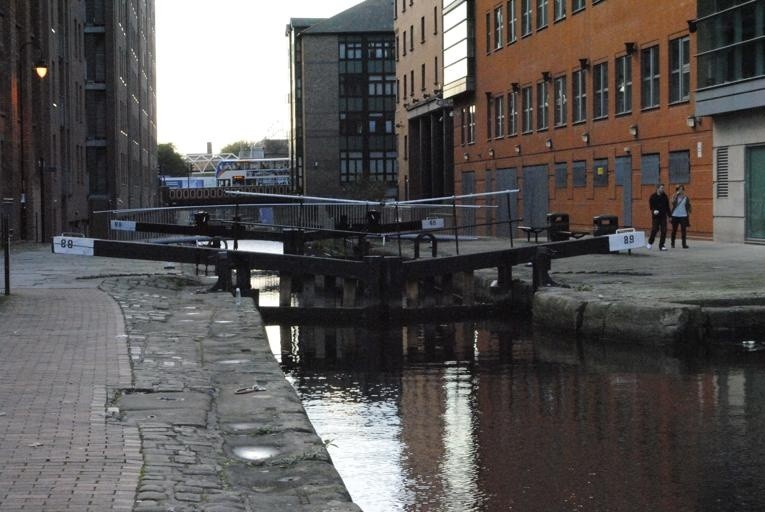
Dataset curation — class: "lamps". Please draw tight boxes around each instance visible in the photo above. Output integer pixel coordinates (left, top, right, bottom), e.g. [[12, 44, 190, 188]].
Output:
[[629, 124, 638, 137], [581, 132, 590, 144], [686, 116, 702, 128], [515, 145, 521, 152], [463, 153, 469, 160], [545, 138, 552, 148], [488, 148, 494, 158]]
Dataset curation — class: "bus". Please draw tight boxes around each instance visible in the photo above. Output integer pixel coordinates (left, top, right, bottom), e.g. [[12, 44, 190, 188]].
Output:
[[216, 158, 291, 187]]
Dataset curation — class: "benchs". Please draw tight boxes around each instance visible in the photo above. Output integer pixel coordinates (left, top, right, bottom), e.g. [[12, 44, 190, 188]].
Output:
[[518, 224, 550, 243], [558, 230, 590, 240]]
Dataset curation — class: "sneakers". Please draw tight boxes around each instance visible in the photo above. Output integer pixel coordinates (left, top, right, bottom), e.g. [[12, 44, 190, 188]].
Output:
[[660, 247, 668, 251], [646, 242, 652, 250]]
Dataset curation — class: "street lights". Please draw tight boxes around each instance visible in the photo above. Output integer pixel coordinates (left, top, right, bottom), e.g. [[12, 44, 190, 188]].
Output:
[[17, 40, 48, 241]]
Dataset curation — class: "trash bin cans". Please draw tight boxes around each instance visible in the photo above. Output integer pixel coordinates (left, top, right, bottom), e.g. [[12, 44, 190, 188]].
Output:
[[593, 216, 617, 233], [547, 213, 569, 241], [195, 211, 209, 226]]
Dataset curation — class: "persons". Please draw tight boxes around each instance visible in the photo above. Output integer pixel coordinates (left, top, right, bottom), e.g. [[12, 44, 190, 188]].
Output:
[[646, 183, 673, 251], [671, 184, 692, 248]]
